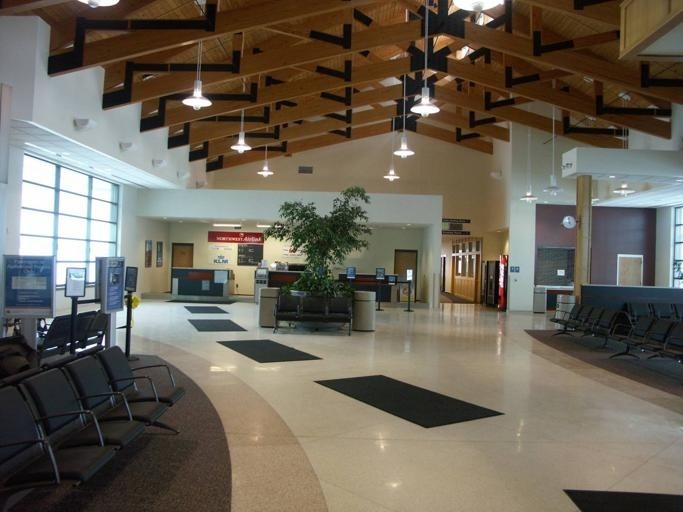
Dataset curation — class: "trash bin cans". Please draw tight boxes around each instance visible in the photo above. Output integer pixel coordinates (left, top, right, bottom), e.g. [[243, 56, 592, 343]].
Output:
[[555, 294, 577, 330], [353, 291, 376, 332], [259, 287, 281, 328]]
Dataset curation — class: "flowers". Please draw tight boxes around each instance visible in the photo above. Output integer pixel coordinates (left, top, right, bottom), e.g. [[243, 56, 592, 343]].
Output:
[[123, 294, 141, 309]]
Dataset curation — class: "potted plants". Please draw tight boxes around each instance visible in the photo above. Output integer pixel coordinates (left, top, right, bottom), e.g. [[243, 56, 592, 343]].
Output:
[[260, 182, 373, 327]]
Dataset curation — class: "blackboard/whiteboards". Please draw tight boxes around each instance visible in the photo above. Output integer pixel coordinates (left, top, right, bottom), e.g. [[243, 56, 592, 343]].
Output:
[[237, 243, 263, 266]]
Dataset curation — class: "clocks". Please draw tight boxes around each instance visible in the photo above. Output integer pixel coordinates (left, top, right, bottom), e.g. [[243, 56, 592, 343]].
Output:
[[561, 215, 576, 229]]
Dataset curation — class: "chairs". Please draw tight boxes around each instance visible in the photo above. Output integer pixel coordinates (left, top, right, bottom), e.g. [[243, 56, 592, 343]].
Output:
[[0, 308, 183, 500], [547, 300, 682, 362], [273, 295, 352, 337]]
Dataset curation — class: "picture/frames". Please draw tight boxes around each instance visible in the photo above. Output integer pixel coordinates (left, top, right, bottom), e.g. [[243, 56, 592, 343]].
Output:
[[614, 253, 643, 286]]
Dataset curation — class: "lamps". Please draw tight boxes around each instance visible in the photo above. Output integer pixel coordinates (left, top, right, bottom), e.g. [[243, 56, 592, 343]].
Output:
[[408, 0, 441, 118], [542, 103, 563, 197], [73, 117, 97, 132], [520, 124, 538, 204], [180, 39, 214, 111], [611, 94, 636, 195], [393, 74, 415, 159], [229, 26, 252, 156], [120, 142, 135, 152], [152, 159, 166, 168], [383, 133, 399, 183], [257, 72, 274, 178]]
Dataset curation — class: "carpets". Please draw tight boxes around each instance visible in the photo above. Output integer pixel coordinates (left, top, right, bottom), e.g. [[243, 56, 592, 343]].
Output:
[[522, 328, 682, 398], [186, 318, 248, 332], [215, 338, 323, 364], [183, 305, 229, 314], [312, 374, 506, 429], [0, 353, 231, 511], [560, 487, 682, 511], [164, 297, 236, 305]]
